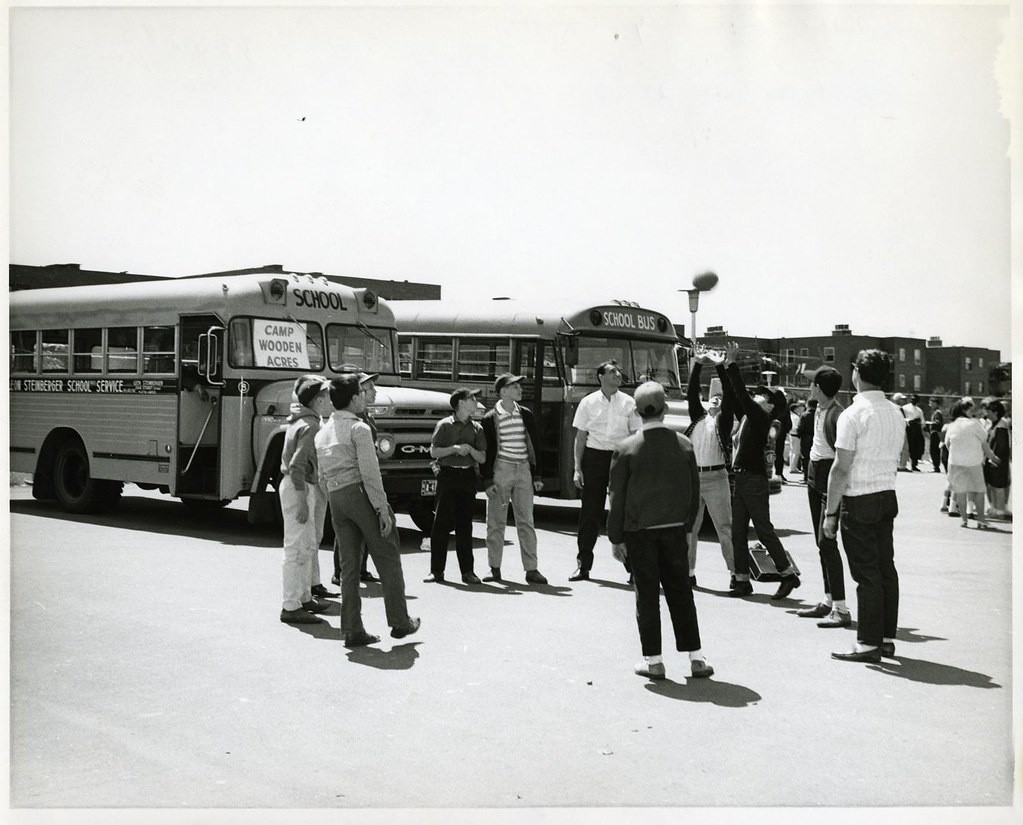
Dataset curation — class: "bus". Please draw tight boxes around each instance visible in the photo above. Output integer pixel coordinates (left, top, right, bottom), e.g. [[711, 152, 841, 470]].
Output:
[[11, 271, 487, 521], [285, 295, 693, 501]]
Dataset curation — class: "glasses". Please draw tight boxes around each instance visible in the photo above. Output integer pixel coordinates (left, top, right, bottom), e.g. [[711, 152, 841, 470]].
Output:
[[463, 395, 477, 402], [356, 387, 367, 394]]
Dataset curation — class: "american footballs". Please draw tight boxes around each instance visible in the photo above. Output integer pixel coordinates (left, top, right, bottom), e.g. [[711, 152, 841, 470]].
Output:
[[692, 271, 719, 292]]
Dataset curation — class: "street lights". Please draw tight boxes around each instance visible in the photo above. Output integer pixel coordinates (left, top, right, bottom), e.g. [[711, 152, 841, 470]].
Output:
[[677, 289, 705, 354]]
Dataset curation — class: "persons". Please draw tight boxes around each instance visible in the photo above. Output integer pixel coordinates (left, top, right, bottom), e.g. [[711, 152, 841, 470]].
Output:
[[607, 382, 714, 678], [147, 334, 210, 402], [683, 340, 851, 626], [822, 347, 906, 663], [891, 392, 1013, 528], [278, 372, 421, 647], [480, 372, 546, 584], [423, 389, 486, 584], [568, 361, 640, 584]]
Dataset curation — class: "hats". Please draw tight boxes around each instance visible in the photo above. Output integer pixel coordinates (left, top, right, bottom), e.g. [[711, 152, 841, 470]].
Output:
[[804, 364, 844, 389], [494, 374, 528, 393], [451, 387, 481, 407], [634, 382, 666, 415], [357, 371, 381, 384], [298, 379, 333, 407]]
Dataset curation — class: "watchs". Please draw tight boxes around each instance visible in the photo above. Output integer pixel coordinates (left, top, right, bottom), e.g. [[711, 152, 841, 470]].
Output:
[[823, 511, 838, 517]]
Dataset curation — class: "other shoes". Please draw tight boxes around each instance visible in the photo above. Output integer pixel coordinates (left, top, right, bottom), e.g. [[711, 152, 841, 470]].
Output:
[[360, 572, 379, 582], [391, 617, 421, 638], [462, 571, 480, 583], [280, 609, 321, 624], [796, 603, 835, 617], [301, 597, 331, 611], [331, 576, 342, 585], [949, 509, 963, 516], [832, 643, 895, 662], [689, 575, 696, 585], [633, 659, 665, 679], [690, 656, 714, 677], [423, 572, 444, 582], [482, 571, 501, 582], [568, 568, 589, 581], [525, 570, 547, 584], [730, 580, 753, 598], [978, 522, 996, 529], [346, 631, 380, 647], [773, 575, 801, 599], [816, 611, 851, 627], [311, 584, 341, 597]]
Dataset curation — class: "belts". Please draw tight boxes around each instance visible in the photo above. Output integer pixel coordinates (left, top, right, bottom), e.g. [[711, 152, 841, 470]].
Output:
[[697, 464, 725, 472]]
